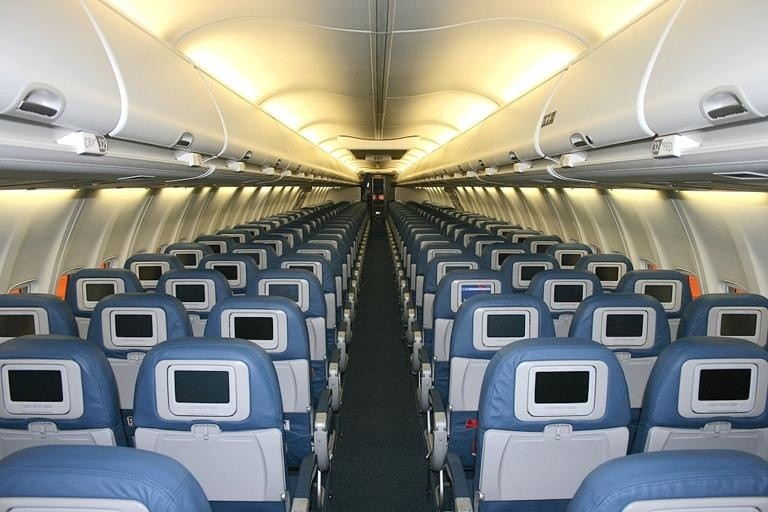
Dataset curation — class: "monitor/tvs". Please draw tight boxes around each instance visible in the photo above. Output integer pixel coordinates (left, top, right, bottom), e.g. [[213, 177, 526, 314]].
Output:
[[642, 282, 676, 309], [0, 310, 40, 344], [601, 310, 647, 346], [230, 312, 279, 350], [715, 310, 761, 345], [2, 365, 71, 414], [424, 214, 627, 310], [527, 366, 596, 416], [482, 311, 530, 347], [167, 365, 236, 417], [110, 310, 158, 347], [692, 364, 758, 413], [66, 218, 334, 309]]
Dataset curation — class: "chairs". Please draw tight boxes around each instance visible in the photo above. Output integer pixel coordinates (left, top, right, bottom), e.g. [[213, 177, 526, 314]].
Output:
[[1, 199, 369, 512], [384, 197, 766, 512]]
